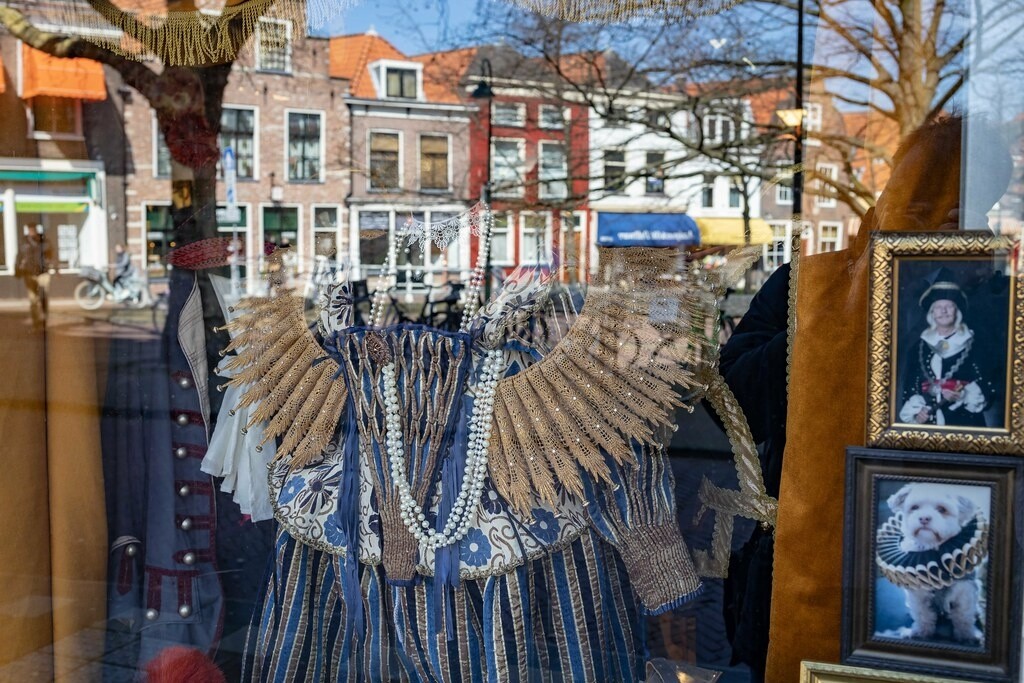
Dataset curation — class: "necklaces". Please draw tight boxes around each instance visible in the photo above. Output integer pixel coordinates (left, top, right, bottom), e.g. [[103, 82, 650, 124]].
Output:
[[365, 195, 540, 551]]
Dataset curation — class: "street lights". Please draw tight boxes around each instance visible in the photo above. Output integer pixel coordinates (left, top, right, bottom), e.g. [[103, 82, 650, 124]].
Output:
[[463, 57, 497, 306]]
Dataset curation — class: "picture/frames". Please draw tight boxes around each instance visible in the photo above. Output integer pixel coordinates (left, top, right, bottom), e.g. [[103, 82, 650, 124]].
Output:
[[868, 230, 1024, 456], [837, 445, 1024, 683]]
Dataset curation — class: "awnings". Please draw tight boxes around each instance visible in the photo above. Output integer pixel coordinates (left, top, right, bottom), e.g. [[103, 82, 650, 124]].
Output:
[[695, 215, 784, 249], [12, 34, 108, 104], [596, 213, 698, 249]]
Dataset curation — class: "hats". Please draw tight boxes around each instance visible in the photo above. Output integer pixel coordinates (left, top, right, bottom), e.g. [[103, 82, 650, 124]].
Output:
[[918, 282, 968, 316]]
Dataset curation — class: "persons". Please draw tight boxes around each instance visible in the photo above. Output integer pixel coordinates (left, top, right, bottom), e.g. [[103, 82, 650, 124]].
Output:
[[17, 222, 54, 331], [208, 158, 698, 683], [102, 241, 137, 309], [670, 113, 1019, 682], [900, 280, 997, 427]]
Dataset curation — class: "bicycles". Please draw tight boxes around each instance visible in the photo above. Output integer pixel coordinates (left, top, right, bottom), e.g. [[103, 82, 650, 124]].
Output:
[[76, 265, 148, 310]]
